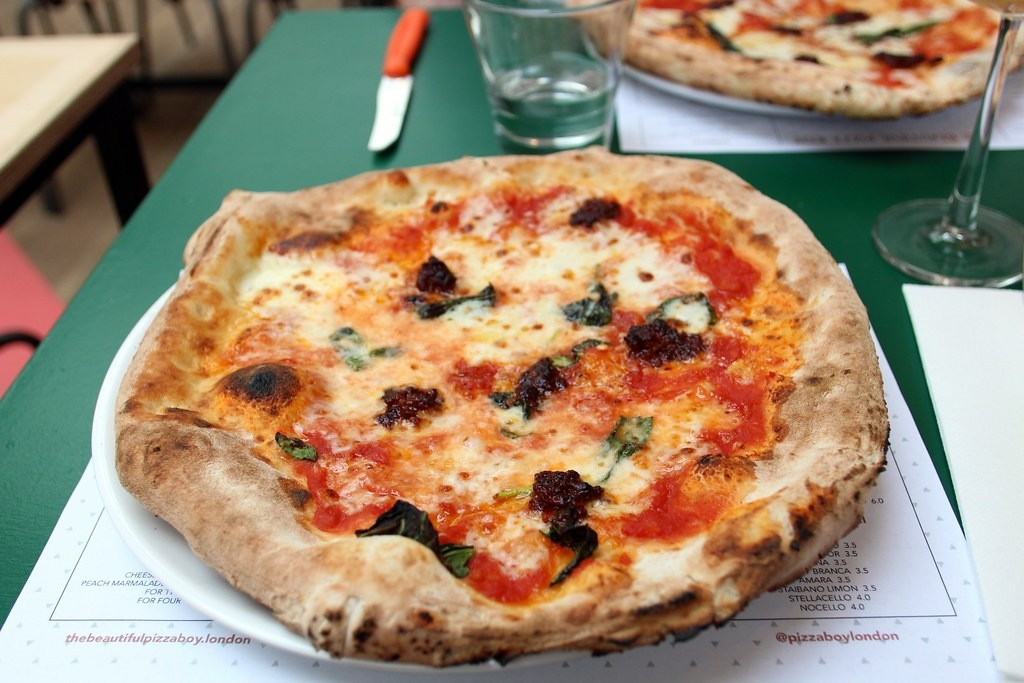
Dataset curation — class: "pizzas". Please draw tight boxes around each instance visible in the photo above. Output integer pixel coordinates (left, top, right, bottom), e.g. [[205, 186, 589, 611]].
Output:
[[563, 0, 1024, 118], [114, 146, 891, 662]]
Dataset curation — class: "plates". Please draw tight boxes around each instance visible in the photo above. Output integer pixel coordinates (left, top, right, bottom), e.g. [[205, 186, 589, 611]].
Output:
[[582, 24, 828, 120], [92, 273, 596, 672]]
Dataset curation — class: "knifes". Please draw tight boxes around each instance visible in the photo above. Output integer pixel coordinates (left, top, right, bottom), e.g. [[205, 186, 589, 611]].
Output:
[[367, 6, 430, 152]]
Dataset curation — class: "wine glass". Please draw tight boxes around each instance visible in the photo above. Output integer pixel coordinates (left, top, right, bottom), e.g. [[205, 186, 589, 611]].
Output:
[[870, 0, 1024, 289]]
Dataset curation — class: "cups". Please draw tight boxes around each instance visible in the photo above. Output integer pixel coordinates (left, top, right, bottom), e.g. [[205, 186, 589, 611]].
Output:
[[461, 0, 637, 151]]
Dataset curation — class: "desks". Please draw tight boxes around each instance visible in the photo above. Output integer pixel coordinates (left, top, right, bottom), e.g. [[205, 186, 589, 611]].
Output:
[[0, 0, 1024, 683], [0, 31, 153, 229]]
